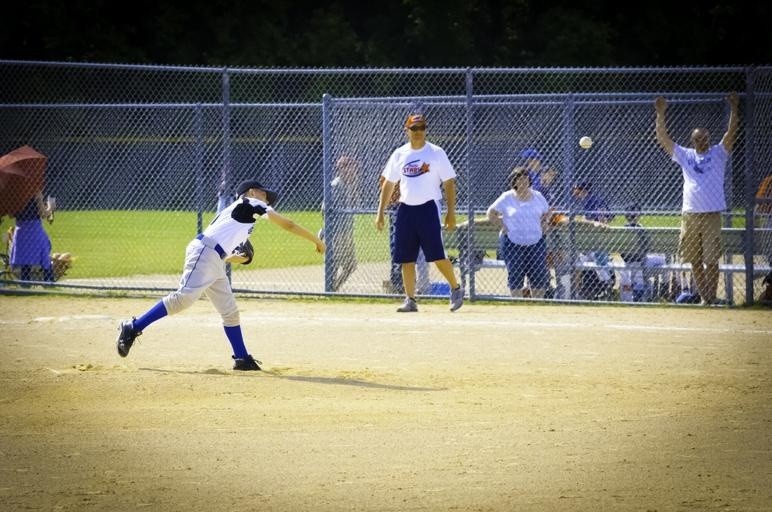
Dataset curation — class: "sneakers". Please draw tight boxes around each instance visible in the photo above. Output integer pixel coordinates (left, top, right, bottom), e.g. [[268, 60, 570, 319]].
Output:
[[232, 355, 260, 372], [117, 317, 141, 357], [397, 298, 417, 311], [449, 286, 465, 310]]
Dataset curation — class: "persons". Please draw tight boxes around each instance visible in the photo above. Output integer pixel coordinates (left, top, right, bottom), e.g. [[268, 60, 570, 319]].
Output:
[[117, 179, 328, 372], [376, 114, 465, 311], [381, 141, 432, 295], [754, 174, 771, 303], [486, 147, 700, 304], [10, 186, 58, 290], [652, 92, 740, 306], [319, 155, 364, 291], [8, 226, 70, 277]]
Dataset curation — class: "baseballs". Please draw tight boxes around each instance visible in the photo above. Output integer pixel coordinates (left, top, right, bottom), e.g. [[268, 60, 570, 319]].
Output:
[[580, 137, 593, 149]]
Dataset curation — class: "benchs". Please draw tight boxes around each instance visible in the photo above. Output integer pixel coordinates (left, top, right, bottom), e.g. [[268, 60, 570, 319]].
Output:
[[415, 217, 772, 307]]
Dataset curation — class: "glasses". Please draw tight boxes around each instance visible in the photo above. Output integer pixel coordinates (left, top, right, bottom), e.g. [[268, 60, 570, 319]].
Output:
[[408, 126, 425, 131]]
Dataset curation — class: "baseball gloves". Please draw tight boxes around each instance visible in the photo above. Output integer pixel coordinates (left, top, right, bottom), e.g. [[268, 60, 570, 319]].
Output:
[[233, 239, 254, 264]]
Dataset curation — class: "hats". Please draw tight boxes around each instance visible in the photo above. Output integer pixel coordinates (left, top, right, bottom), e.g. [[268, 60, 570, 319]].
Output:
[[407, 115, 425, 125], [338, 157, 360, 168], [522, 149, 544, 159], [236, 180, 278, 205]]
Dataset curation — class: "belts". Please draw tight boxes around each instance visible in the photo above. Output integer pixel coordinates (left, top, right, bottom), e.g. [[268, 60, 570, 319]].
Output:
[[196, 234, 227, 259]]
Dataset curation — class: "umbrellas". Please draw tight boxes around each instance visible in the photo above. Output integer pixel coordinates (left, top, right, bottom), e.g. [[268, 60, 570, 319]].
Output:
[[0, 145, 49, 218]]
